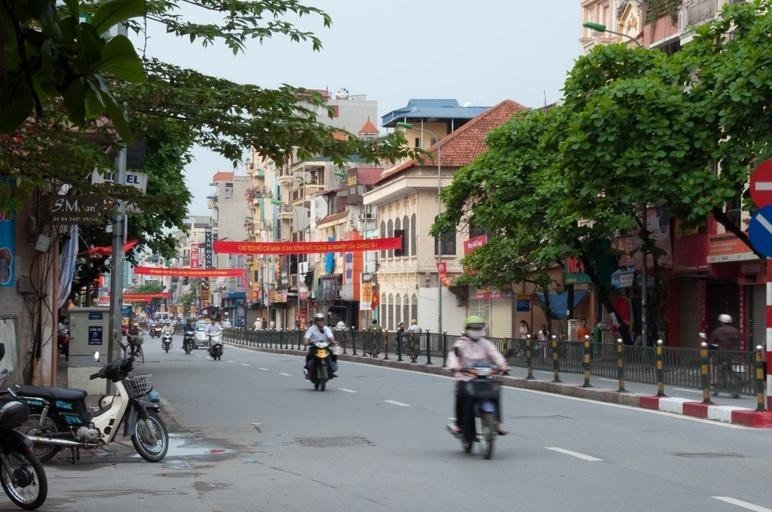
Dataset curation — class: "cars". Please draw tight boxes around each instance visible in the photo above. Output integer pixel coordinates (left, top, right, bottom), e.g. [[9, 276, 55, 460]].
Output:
[[192, 319, 215, 349]]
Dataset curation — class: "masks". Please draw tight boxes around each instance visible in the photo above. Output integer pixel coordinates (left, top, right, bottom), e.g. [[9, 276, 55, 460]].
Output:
[[466, 329, 482, 339]]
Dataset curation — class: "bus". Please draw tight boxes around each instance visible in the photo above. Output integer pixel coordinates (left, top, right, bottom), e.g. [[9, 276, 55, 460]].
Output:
[[120, 303, 133, 336]]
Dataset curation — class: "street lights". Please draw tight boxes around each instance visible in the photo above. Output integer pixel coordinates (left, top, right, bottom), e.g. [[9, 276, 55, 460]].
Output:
[[581, 18, 644, 48], [396, 120, 444, 351], [269, 197, 302, 315], [333, 170, 369, 328]]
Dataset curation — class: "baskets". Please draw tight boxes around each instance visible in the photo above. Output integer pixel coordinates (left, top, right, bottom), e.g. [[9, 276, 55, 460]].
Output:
[[464, 381, 500, 400], [1, 398, 29, 430]]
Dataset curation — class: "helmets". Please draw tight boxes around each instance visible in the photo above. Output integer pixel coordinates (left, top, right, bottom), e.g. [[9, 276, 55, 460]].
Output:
[[314, 312, 325, 318], [463, 316, 485, 328]]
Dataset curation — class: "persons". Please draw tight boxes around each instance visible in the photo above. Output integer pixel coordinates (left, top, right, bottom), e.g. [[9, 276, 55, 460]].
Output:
[[538, 324, 549, 357], [253, 317, 267, 331], [398, 319, 421, 359], [336, 318, 346, 331], [160, 321, 174, 346], [58, 316, 69, 335], [578, 319, 591, 341], [125, 319, 146, 355], [224, 319, 231, 328], [205, 318, 223, 351], [519, 320, 530, 340], [183, 319, 194, 333], [447, 315, 511, 440], [304, 313, 339, 378], [706, 310, 743, 400], [368, 319, 382, 355]]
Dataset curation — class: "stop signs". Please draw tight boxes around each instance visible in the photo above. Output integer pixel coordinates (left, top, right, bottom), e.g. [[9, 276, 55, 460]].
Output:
[[749, 157, 772, 208]]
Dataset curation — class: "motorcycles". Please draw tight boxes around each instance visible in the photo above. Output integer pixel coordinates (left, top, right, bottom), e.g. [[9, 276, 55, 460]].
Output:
[[0, 343, 49, 511], [0, 335, 170, 465], [124, 311, 225, 363], [303, 338, 339, 392], [698, 331, 747, 401], [444, 345, 513, 462], [404, 339, 420, 364]]
